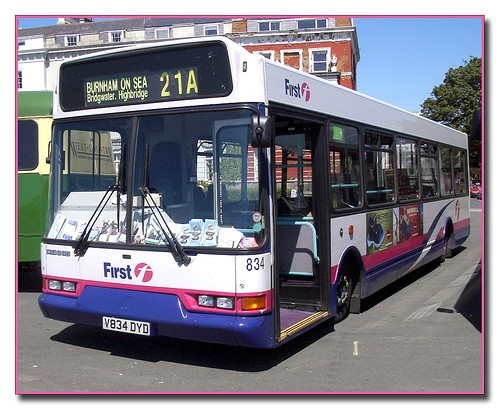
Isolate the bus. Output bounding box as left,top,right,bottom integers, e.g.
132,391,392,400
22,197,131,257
39,37,470,349
18,91,119,267
